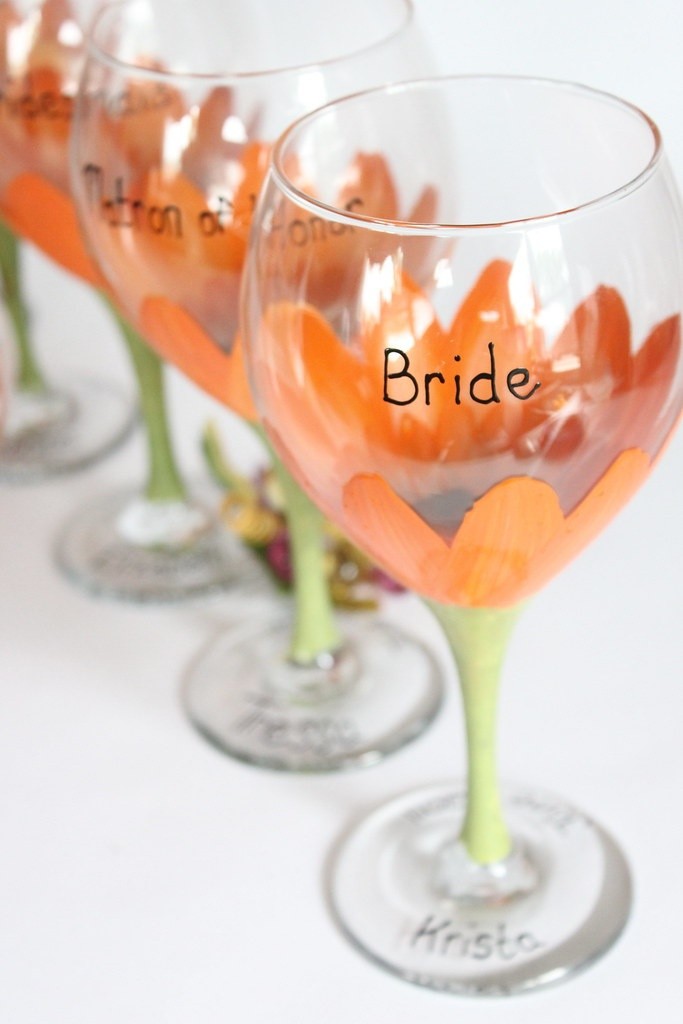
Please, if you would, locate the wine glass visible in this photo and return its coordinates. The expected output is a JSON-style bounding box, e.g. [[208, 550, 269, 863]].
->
[[71, 0, 445, 774], [1, 0, 251, 603], [235, 72, 683, 1000], [0, 0, 137, 484]]
